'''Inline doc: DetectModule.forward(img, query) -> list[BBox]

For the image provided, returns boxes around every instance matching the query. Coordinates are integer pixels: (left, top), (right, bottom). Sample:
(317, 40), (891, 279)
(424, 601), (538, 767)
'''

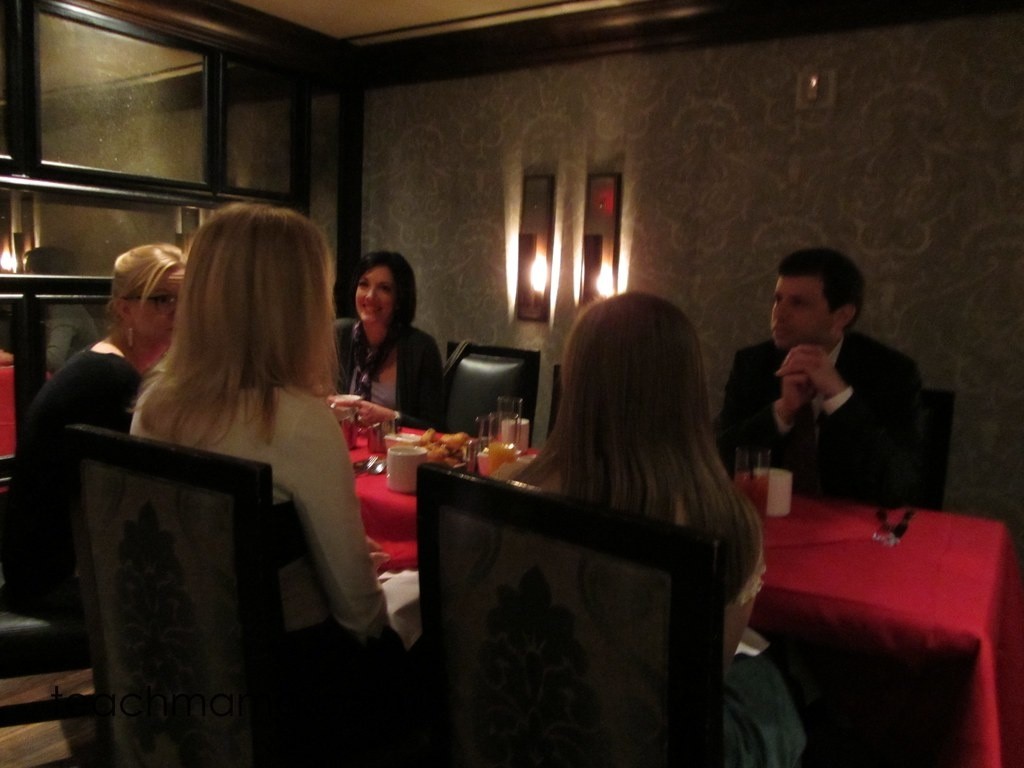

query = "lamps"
(0, 192), (36, 277)
(512, 169), (556, 323)
(576, 173), (622, 313)
(177, 207), (201, 255)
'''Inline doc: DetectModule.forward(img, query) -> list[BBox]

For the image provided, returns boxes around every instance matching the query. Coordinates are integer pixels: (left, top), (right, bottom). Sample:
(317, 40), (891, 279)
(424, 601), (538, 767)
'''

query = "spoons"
(369, 461), (387, 474)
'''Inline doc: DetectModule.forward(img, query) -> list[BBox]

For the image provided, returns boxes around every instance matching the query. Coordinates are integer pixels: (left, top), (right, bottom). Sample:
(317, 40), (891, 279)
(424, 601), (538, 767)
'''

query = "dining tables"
(346, 424), (1024, 768)
(440, 342), (541, 450)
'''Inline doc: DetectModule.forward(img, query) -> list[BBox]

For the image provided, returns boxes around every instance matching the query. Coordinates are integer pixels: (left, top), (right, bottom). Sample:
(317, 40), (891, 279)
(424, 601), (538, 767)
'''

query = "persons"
(710, 246), (925, 507)
(2, 242), (187, 621)
(125, 203), (409, 768)
(332, 249), (448, 433)
(511, 290), (821, 768)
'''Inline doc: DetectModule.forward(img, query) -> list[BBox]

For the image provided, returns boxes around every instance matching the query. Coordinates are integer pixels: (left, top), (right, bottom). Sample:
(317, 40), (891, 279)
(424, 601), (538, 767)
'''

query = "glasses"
(120, 294), (178, 311)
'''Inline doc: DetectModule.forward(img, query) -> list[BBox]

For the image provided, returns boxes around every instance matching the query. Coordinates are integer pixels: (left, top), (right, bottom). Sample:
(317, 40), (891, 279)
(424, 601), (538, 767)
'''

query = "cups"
(479, 397), (529, 453)
(388, 446), (428, 493)
(736, 446), (771, 517)
(327, 394), (363, 450)
(753, 468), (792, 517)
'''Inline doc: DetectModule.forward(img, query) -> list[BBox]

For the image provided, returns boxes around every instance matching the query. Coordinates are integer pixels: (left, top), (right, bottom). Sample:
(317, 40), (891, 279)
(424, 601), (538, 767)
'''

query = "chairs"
(416, 462), (729, 768)
(0, 454), (99, 730)
(64, 422), (285, 768)
(911, 389), (955, 511)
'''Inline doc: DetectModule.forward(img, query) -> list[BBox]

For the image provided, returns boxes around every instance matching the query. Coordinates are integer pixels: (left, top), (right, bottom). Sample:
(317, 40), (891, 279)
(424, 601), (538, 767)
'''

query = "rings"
(330, 403), (335, 410)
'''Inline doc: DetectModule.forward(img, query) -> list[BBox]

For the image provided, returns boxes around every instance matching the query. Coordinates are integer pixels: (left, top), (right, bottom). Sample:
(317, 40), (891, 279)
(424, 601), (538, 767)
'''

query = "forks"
(352, 456), (378, 478)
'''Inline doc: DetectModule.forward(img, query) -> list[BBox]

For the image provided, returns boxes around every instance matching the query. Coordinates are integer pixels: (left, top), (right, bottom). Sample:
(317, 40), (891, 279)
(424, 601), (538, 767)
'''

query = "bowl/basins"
(384, 435), (422, 453)
